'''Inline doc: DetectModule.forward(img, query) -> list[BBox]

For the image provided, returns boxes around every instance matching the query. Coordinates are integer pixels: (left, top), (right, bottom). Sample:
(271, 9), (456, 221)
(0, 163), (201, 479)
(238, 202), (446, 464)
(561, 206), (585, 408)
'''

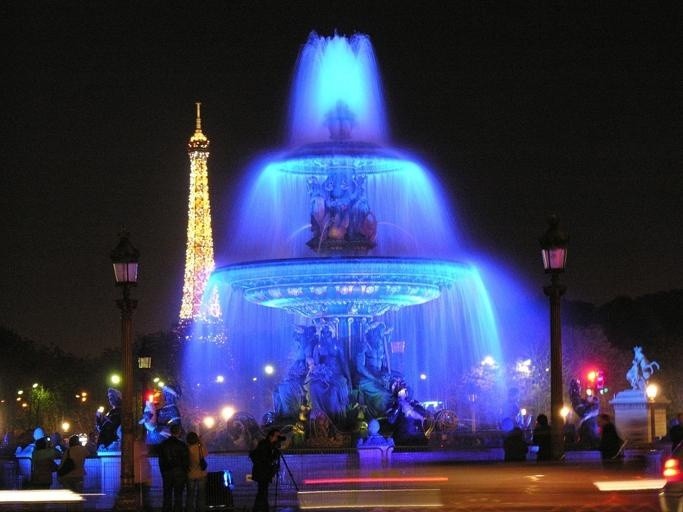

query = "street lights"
(534, 210), (572, 465)
(465, 384), (479, 433)
(134, 340), (152, 442)
(106, 231), (153, 512)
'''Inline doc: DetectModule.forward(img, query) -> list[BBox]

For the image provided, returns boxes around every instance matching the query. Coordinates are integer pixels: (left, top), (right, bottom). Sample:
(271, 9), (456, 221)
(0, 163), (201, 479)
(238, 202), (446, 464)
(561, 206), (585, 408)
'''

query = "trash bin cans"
(207, 473), (233, 508)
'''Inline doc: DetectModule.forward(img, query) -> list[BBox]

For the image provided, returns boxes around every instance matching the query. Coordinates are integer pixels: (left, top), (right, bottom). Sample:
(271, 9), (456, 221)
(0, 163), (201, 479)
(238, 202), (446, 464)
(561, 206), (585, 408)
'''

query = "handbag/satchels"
(199, 457), (208, 471)
(50, 461), (58, 472)
(55, 463), (85, 484)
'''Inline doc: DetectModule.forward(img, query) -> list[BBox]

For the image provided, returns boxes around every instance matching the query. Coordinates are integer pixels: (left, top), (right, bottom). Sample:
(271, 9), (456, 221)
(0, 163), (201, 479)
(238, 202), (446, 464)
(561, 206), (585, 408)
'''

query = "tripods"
(271, 445), (299, 512)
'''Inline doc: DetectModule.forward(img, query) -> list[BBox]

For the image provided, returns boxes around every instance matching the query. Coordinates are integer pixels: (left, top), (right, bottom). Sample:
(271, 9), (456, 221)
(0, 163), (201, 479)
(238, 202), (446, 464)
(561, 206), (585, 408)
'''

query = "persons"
(598, 415), (624, 472)
(571, 378), (597, 437)
(502, 388), (520, 428)
(503, 418), (514, 432)
(158, 424), (189, 512)
(249, 429), (280, 512)
(262, 322), (433, 447)
(503, 427), (529, 462)
(143, 384), (181, 455)
(0, 428), (98, 512)
(96, 388), (123, 453)
(186, 432), (209, 512)
(669, 414), (683, 455)
(533, 415), (551, 462)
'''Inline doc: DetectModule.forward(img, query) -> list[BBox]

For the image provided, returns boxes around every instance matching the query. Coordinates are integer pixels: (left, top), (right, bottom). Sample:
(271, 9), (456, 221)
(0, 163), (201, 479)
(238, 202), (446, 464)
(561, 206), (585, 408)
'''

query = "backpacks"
(172, 440), (191, 469)
(544, 426), (565, 459)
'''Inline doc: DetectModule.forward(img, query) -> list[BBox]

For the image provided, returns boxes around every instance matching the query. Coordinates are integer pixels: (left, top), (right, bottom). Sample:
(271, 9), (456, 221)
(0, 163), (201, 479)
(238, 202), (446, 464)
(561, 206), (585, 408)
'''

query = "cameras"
(275, 435), (288, 444)
